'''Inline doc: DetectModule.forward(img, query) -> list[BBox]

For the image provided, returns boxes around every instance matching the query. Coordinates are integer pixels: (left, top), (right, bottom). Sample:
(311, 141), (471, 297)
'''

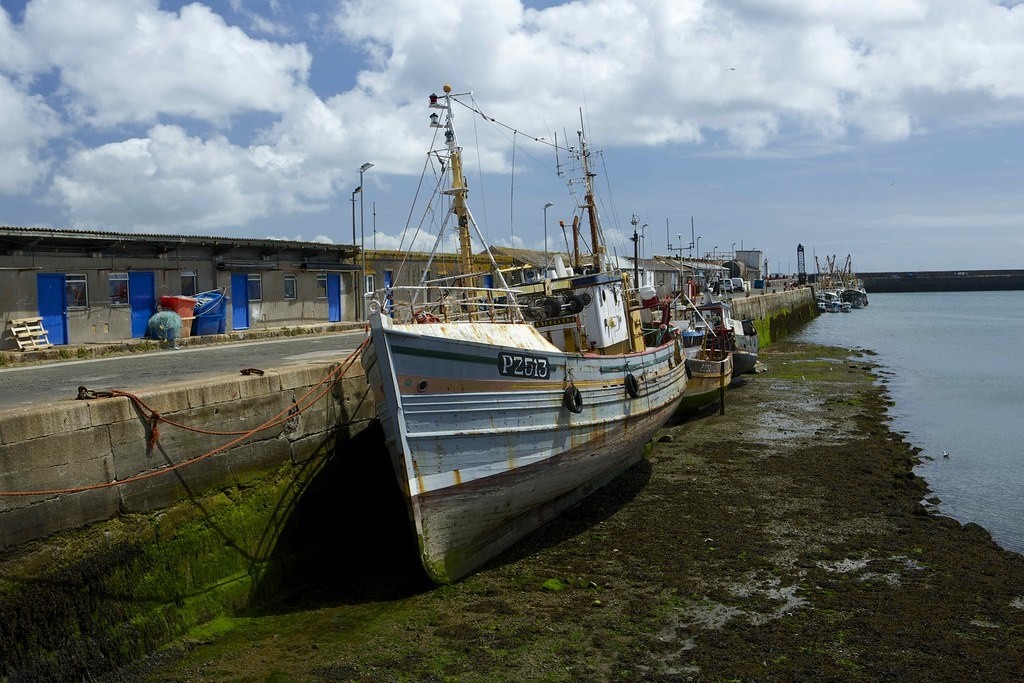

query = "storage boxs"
(672, 320), (690, 330)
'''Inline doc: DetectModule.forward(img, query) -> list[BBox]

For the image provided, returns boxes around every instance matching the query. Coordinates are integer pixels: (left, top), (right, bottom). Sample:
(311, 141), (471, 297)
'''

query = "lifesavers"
(564, 386), (584, 414)
(624, 373), (640, 399)
(685, 359), (692, 380)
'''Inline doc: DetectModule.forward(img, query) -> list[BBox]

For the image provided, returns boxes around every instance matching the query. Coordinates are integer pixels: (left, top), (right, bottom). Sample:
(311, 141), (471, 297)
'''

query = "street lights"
(544, 202), (554, 278)
(360, 163), (375, 321)
(732, 243), (736, 259)
(641, 223), (648, 259)
(353, 186), (364, 321)
(714, 246), (718, 260)
(697, 236), (702, 271)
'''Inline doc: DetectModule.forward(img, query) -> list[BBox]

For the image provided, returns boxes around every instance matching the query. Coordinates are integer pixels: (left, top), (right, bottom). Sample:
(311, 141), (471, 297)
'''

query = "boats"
(815, 255), (868, 313)
(358, 83), (692, 590)
(668, 285), (760, 382)
(666, 316), (735, 424)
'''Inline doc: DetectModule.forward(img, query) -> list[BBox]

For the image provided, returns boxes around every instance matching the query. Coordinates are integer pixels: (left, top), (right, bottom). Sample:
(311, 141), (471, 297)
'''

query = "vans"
(719, 278), (734, 294)
(732, 277), (747, 292)
